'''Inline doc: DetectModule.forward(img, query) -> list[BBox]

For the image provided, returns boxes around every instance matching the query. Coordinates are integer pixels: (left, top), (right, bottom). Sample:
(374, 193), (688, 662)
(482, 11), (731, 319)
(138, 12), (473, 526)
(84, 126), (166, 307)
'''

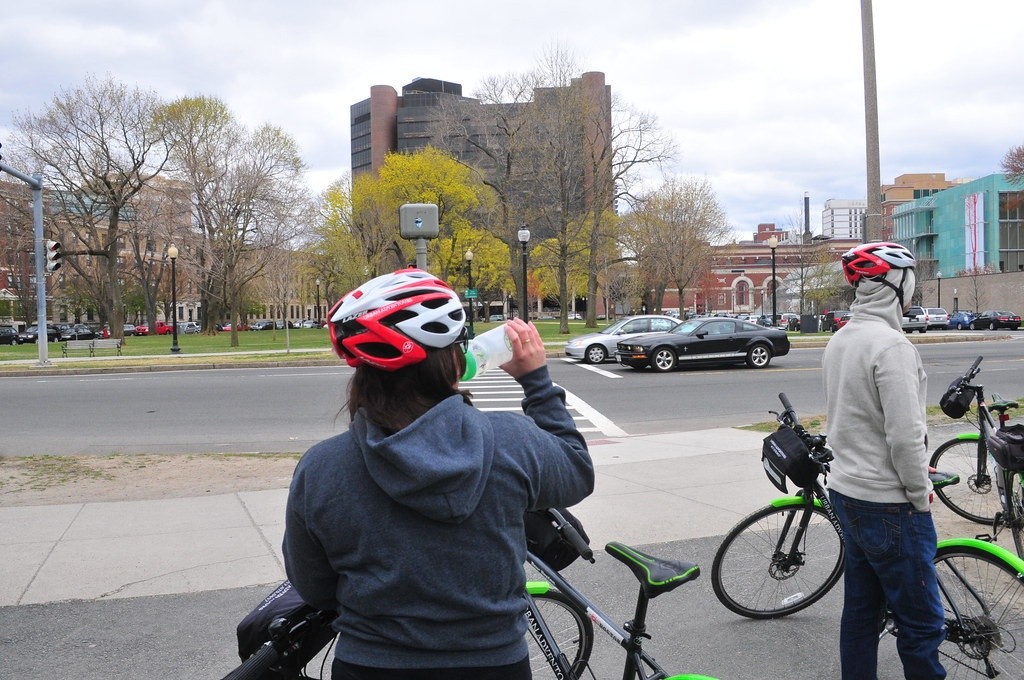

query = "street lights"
(936, 270), (942, 308)
(167, 243), (181, 352)
(123, 303), (127, 323)
(518, 219), (530, 323)
(766, 233), (779, 327)
(315, 278), (320, 330)
(464, 248), (475, 339)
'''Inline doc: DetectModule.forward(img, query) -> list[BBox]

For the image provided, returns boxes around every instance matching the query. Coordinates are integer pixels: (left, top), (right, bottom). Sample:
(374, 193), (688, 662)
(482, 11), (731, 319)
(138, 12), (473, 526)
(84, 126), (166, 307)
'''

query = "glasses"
(452, 326), (468, 353)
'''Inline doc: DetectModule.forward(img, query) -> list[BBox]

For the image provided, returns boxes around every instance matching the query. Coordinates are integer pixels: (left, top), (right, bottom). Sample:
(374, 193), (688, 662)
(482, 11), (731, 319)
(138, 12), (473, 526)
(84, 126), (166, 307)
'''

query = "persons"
(626, 320), (644, 333)
(822, 241), (947, 680)
(281, 266), (594, 680)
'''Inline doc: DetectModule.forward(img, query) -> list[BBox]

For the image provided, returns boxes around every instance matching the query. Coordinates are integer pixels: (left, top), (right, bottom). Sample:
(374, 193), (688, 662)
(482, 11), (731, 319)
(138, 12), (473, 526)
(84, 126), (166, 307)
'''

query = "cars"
(614, 316), (790, 373)
(275, 319), (293, 329)
(672, 310), (852, 334)
(52, 321), (110, 341)
(0, 325), (20, 345)
(970, 310), (1021, 331)
(250, 319), (277, 331)
(482, 314), (503, 322)
(177, 320), (249, 334)
(293, 319), (328, 329)
(537, 312), (605, 320)
(947, 311), (975, 331)
(123, 324), (136, 337)
(135, 321), (174, 336)
(17, 323), (62, 344)
(565, 314), (695, 365)
(923, 307), (949, 331)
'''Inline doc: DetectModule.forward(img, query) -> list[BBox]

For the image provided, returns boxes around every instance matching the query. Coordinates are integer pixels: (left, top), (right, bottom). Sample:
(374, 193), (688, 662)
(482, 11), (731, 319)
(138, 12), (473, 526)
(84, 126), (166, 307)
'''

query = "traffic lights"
(46, 239), (62, 273)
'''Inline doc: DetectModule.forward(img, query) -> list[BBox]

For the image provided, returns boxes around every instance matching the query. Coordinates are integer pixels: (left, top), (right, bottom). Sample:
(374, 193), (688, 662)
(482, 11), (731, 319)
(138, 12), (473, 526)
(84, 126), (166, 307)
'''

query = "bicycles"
(928, 355), (1023, 559)
(524, 506), (719, 680)
(711, 392), (1024, 680)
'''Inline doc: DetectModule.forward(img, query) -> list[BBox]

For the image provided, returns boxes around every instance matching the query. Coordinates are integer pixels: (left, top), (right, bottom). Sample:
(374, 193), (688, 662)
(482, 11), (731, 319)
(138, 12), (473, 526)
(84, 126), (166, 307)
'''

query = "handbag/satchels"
(238, 578), (305, 661)
(988, 424), (1024, 469)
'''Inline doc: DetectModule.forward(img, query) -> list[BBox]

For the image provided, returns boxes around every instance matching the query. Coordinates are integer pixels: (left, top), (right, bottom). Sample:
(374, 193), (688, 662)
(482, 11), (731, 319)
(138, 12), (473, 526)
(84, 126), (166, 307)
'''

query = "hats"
(328, 270), (466, 370)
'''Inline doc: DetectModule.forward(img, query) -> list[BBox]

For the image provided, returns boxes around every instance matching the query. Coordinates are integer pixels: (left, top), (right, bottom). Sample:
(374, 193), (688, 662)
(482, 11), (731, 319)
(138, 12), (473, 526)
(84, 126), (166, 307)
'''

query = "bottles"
(460, 323), (530, 382)
(997, 462), (1005, 490)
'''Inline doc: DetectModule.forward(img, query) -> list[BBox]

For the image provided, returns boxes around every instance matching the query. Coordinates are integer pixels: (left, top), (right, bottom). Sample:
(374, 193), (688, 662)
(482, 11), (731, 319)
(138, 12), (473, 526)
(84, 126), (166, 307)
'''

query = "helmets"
(841, 243), (916, 288)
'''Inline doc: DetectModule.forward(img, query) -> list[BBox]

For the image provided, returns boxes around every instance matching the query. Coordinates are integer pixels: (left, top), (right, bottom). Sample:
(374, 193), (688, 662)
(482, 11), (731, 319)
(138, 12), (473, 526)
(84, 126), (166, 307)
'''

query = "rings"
(522, 338), (530, 343)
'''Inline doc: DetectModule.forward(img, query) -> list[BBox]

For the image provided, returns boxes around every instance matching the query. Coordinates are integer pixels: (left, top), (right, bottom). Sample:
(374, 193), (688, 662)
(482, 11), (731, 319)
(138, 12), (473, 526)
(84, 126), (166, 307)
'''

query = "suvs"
(901, 305), (930, 334)
